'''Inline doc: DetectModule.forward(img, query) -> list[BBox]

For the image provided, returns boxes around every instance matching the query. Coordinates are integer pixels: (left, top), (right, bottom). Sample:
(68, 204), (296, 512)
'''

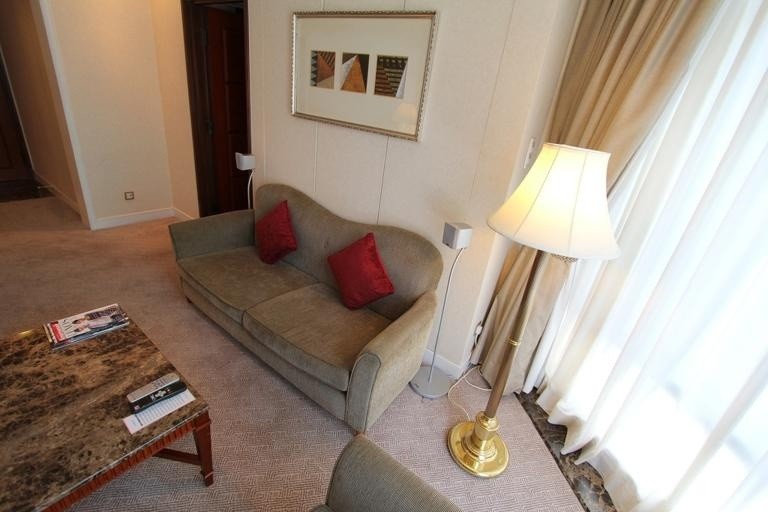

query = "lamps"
(235, 152), (257, 212)
(444, 143), (625, 478)
(406, 221), (474, 398)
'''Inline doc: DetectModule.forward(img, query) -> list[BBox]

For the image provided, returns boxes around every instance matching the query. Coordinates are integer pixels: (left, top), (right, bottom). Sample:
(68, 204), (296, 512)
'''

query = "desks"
(0, 301), (216, 512)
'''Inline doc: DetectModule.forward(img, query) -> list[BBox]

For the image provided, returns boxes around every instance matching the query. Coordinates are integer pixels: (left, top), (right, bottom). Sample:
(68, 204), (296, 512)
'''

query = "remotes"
(129, 382), (186, 412)
(127, 373), (180, 404)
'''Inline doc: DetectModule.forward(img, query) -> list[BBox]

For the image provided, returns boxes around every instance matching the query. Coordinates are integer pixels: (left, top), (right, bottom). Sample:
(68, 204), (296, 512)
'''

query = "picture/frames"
(288, 7), (441, 143)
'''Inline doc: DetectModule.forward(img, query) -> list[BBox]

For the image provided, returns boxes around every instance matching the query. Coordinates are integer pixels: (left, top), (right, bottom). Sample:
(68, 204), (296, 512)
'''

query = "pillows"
(255, 199), (297, 265)
(325, 230), (394, 310)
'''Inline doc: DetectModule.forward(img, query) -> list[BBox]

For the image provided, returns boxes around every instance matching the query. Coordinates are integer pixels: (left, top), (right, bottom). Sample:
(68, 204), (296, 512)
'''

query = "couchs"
(300, 432), (465, 511)
(166, 181), (447, 441)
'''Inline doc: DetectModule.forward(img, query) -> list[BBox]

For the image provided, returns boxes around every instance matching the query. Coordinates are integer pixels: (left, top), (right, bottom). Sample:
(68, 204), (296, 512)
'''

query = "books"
(42, 303), (130, 350)
